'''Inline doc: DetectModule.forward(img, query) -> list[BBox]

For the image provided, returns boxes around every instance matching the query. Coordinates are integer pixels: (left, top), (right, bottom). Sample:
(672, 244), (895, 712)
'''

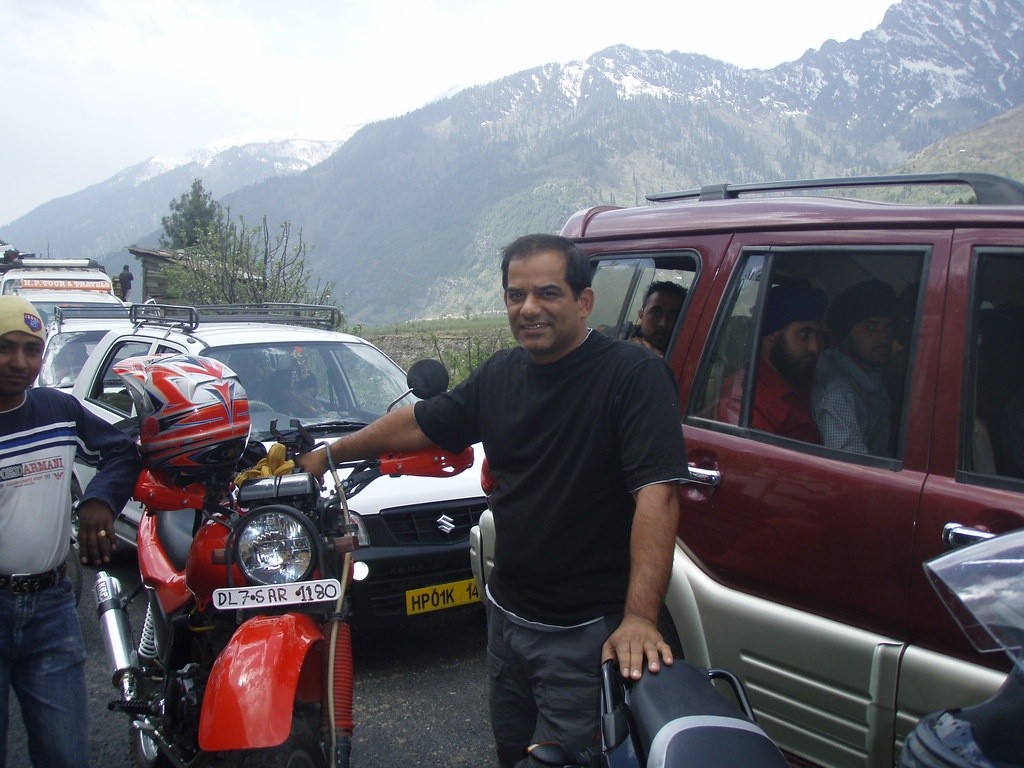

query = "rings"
(98, 530), (105, 536)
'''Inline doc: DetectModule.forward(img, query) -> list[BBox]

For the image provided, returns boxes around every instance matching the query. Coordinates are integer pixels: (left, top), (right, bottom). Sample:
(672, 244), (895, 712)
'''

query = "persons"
(295, 234), (692, 768)
(0, 265), (919, 768)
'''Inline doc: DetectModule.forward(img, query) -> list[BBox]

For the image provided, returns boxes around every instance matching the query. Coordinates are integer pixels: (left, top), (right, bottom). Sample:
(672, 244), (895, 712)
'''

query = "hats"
(761, 286), (827, 335)
(0, 295), (46, 344)
(828, 280), (897, 338)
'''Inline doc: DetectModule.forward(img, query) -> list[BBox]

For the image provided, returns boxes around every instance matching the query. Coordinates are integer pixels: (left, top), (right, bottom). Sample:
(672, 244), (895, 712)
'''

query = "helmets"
(898, 529), (1023, 768)
(112, 353), (252, 486)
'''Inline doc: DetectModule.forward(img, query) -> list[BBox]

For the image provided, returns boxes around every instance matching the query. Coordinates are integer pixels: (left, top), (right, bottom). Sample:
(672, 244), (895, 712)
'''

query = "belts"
(0, 560), (67, 595)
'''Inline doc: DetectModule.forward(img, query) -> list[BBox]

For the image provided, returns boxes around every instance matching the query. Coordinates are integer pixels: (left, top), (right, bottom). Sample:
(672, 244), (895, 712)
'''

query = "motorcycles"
(90, 357), (479, 768)
(594, 522), (1024, 767)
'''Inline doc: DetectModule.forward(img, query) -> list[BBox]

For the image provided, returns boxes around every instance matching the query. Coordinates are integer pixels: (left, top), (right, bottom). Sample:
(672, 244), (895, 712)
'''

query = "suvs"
(0, 259), (161, 396)
(69, 292), (486, 641)
(470, 171), (1024, 768)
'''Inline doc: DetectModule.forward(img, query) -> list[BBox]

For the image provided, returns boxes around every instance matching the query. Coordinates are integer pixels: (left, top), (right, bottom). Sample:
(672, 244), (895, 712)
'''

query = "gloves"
(233, 443), (295, 488)
